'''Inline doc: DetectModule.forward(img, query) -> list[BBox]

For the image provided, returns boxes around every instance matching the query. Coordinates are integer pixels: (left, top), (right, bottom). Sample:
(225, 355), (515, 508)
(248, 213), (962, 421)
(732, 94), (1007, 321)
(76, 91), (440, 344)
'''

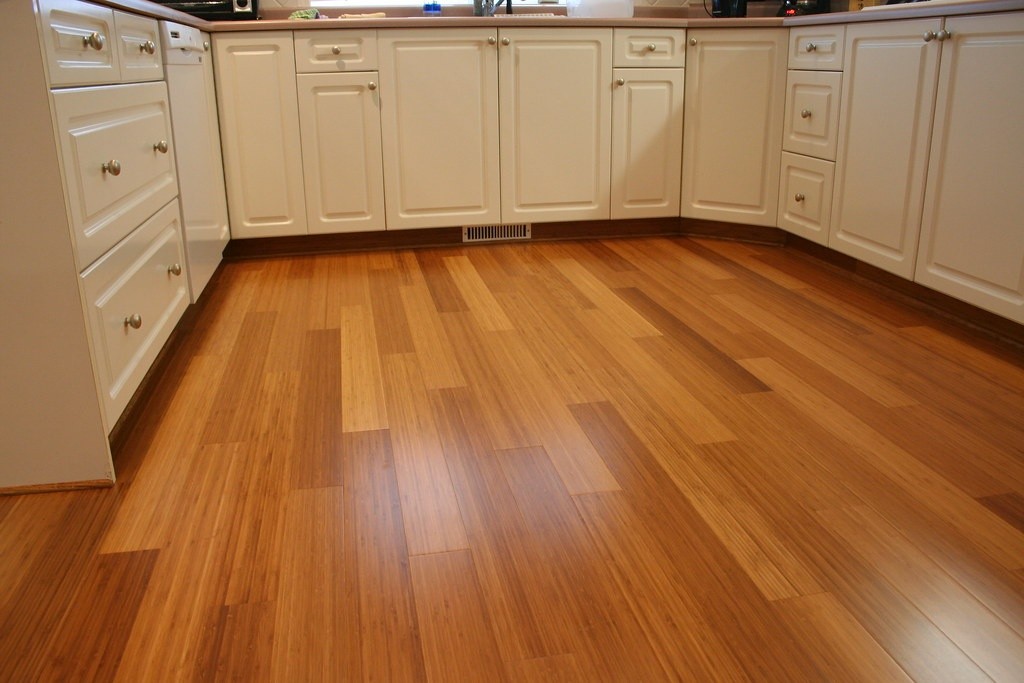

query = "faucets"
(483, 0), (504, 17)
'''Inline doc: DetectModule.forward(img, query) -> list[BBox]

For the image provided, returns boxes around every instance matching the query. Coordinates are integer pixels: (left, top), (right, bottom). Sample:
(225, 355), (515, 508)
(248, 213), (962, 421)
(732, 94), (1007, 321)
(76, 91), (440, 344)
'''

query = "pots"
(703, 0), (749, 18)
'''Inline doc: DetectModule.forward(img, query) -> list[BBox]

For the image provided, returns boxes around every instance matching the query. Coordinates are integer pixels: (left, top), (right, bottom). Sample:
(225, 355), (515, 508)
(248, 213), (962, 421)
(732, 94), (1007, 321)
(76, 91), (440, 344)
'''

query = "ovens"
(152, 0), (259, 23)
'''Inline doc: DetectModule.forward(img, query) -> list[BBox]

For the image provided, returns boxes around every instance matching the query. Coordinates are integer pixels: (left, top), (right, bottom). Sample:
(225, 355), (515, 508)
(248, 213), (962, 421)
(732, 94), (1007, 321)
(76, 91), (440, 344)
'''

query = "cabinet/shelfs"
(377, 25), (612, 250)
(0, 0), (231, 494)
(830, 11), (1024, 346)
(611, 26), (791, 247)
(209, 27), (387, 260)
(777, 25), (846, 261)
